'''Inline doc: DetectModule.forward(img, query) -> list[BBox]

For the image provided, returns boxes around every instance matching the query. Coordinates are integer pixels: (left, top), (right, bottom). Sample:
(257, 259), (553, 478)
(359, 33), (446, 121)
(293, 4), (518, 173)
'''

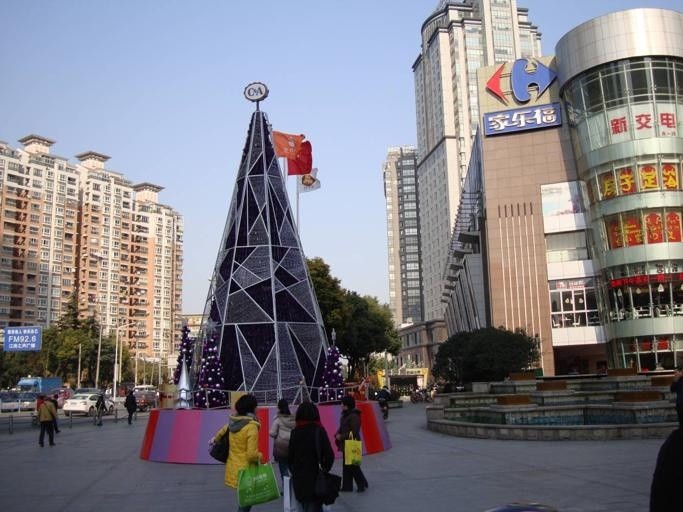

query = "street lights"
(111, 322), (135, 398)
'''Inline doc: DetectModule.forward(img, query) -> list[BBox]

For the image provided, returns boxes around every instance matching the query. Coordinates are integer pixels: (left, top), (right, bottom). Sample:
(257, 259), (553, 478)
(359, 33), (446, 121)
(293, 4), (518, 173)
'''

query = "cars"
(0, 391), (44, 412)
(123, 391), (159, 412)
(62, 393), (114, 417)
(101, 381), (160, 396)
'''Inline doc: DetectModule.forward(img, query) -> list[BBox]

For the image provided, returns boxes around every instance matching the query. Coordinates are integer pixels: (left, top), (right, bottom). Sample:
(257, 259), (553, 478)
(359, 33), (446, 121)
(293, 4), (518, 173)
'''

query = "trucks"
(17, 376), (62, 395)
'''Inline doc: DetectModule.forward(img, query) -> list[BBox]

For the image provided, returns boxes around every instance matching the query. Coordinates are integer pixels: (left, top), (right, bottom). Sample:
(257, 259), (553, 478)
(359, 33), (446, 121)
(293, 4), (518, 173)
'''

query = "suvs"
(44, 387), (73, 408)
(98, 393), (114, 404)
(74, 388), (100, 394)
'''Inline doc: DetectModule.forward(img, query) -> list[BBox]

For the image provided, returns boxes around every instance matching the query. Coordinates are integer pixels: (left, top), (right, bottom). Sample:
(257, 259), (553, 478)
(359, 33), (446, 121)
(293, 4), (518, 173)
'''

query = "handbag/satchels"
(236, 463), (280, 506)
(211, 430), (229, 463)
(344, 440), (363, 465)
(317, 469), (341, 504)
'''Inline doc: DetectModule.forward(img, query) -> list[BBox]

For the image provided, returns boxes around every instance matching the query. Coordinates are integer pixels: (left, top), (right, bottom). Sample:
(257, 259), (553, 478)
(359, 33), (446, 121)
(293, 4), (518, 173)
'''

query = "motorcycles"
(373, 391), (388, 421)
(409, 384), (434, 405)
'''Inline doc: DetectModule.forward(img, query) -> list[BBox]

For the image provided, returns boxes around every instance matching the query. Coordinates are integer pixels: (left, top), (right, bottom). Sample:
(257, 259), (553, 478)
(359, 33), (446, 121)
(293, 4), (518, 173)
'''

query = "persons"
(211, 393), (263, 512)
(270, 398), (298, 497)
(670, 364), (683, 429)
(378, 385), (392, 421)
(35, 392), (60, 447)
(104, 386), (112, 399)
(95, 394), (107, 426)
(334, 396), (369, 496)
(649, 426), (683, 512)
(286, 402), (334, 512)
(124, 390), (137, 425)
(598, 364), (608, 374)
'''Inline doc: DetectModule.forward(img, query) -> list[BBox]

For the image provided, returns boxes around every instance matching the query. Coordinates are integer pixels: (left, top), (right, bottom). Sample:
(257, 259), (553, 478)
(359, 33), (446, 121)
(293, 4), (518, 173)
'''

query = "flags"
(297, 168), (321, 193)
(271, 130), (305, 160)
(286, 140), (312, 175)
